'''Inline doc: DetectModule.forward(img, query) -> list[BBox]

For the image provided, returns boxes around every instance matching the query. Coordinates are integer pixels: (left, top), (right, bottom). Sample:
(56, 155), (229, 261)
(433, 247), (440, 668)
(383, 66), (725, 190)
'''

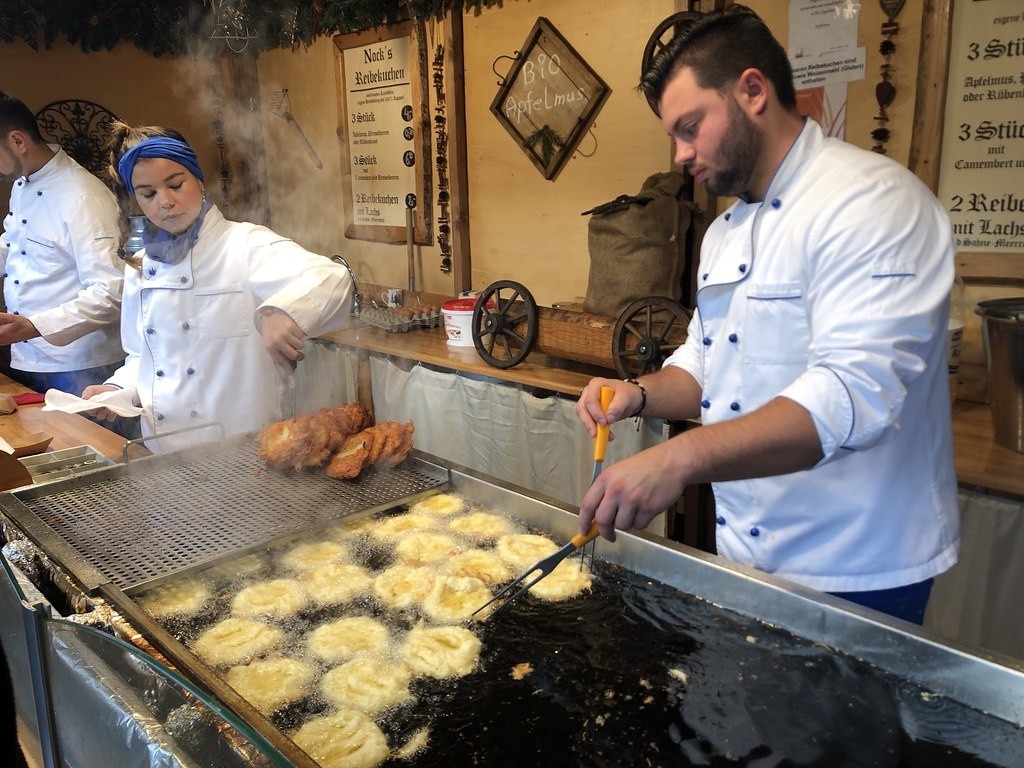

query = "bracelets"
(624, 378), (648, 418)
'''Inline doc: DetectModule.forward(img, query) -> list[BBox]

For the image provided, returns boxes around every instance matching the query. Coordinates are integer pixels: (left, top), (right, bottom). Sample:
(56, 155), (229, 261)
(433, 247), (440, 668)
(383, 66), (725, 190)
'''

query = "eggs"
(393, 303), (441, 320)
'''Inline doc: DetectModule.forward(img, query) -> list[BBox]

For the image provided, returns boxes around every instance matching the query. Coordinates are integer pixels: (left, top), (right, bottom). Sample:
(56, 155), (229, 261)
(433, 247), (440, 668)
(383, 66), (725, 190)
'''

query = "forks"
(473, 521), (602, 624)
(577, 384), (615, 574)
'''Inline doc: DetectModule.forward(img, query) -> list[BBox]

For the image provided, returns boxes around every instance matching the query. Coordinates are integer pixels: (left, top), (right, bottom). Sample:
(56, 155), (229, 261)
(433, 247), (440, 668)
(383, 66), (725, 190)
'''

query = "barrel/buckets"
(441, 299), (494, 346)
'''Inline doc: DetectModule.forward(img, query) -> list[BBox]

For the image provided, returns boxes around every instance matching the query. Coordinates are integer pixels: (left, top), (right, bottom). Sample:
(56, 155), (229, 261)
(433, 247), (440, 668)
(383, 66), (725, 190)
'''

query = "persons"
(577, 5), (961, 627)
(0, 92), (134, 440)
(81, 120), (354, 456)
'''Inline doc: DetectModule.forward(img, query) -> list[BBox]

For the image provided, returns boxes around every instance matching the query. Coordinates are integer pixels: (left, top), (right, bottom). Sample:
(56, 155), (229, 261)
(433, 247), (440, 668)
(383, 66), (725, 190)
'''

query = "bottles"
(947, 270), (966, 408)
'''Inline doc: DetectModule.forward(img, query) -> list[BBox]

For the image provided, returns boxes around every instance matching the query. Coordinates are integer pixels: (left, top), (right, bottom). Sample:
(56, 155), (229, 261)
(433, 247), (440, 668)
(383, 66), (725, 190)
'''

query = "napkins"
(40, 389), (143, 417)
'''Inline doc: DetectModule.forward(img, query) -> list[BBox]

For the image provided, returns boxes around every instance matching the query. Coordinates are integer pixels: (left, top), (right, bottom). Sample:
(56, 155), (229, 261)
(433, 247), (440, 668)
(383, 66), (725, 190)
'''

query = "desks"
(0, 371), (153, 464)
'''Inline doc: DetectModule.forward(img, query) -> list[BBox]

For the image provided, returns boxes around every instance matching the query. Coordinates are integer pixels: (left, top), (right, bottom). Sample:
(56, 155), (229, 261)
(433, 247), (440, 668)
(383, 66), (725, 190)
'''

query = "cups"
(381, 288), (403, 308)
(974, 297), (1024, 453)
